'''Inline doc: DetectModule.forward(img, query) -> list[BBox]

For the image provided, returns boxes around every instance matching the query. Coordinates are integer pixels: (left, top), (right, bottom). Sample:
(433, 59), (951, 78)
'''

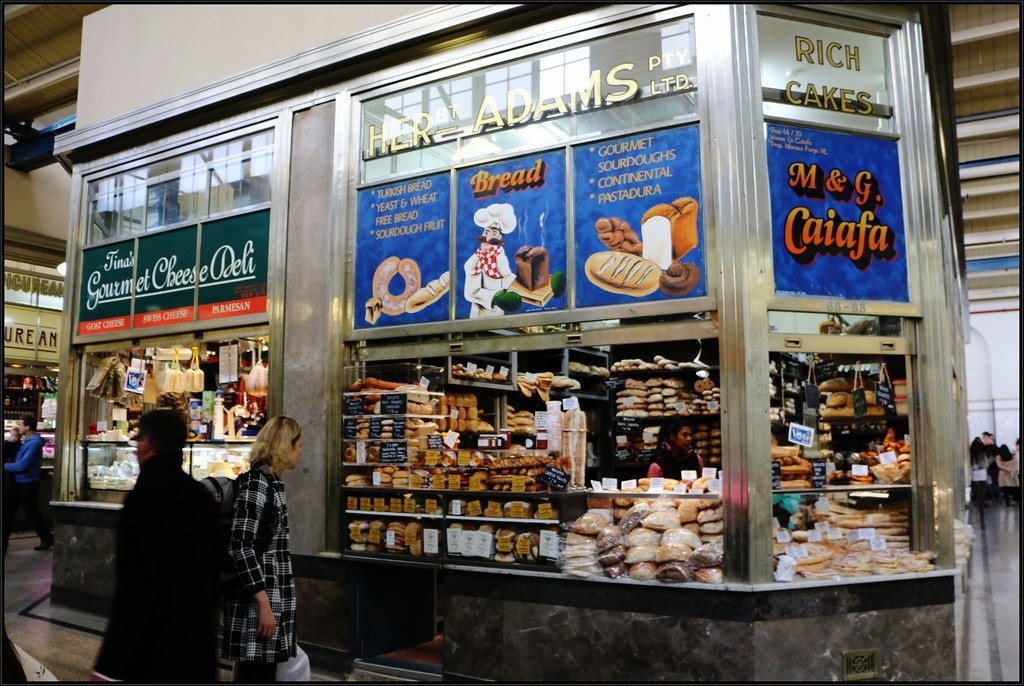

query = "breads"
(342, 354), (726, 584)
(584, 198), (699, 297)
(514, 245), (549, 291)
(365, 256), (450, 325)
(771, 376), (968, 581)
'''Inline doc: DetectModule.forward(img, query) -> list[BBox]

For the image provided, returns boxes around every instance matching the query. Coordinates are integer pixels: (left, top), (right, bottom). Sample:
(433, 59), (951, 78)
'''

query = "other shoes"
(34, 534), (54, 551)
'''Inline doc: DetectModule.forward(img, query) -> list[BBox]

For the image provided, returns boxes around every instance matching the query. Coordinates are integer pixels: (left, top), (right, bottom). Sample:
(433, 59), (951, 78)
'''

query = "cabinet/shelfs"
(336, 320), (912, 567)
(4, 376), (58, 452)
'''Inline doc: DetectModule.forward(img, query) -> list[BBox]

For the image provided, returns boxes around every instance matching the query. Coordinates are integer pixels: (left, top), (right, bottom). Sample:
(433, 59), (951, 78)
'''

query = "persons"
(91, 410), (231, 683)
(2, 415), (55, 554)
(771, 425), (803, 532)
(215, 415), (302, 682)
(647, 418), (703, 479)
(969, 432), (1021, 508)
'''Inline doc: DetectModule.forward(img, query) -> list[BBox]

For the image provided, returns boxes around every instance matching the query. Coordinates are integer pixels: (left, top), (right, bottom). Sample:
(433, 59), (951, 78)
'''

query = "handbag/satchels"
(232, 643), (310, 681)
(198, 470), (274, 574)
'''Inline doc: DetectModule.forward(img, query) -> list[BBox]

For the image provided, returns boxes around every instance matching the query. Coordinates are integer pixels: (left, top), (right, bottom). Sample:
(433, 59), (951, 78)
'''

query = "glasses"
(127, 434), (142, 448)
